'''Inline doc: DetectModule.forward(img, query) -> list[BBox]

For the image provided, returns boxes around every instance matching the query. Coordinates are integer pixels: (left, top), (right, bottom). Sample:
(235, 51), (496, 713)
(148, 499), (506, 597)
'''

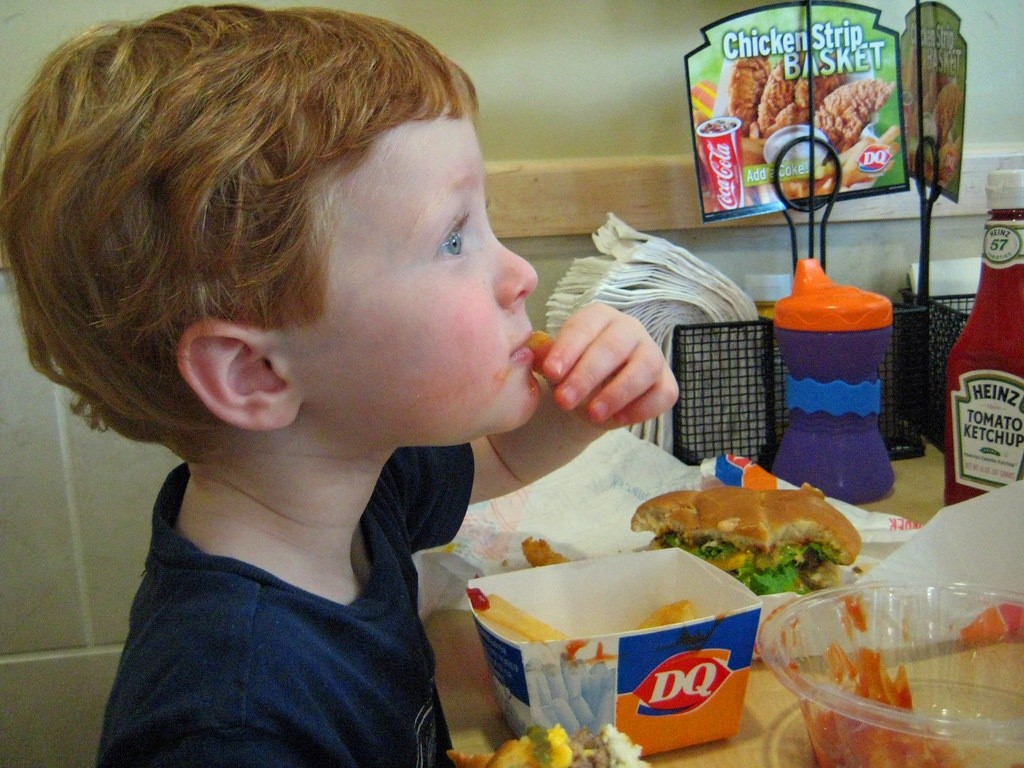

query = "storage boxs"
(465, 547), (763, 756)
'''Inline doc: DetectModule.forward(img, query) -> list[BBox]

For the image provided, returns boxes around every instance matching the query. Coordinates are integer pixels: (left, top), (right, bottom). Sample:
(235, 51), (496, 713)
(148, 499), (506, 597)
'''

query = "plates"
(762, 124), (829, 165)
(918, 112), (938, 145)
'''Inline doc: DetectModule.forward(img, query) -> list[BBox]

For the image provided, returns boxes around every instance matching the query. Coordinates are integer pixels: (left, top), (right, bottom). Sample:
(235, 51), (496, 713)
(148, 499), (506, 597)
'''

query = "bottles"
(945, 155), (1024, 508)
(747, 274), (793, 321)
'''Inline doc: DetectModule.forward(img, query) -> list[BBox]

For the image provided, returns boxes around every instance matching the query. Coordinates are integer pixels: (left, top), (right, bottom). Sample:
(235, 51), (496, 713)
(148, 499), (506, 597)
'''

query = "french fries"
(781, 127), (899, 197)
(476, 595), (566, 644)
(529, 331), (553, 366)
(640, 601), (692, 629)
(523, 537), (569, 567)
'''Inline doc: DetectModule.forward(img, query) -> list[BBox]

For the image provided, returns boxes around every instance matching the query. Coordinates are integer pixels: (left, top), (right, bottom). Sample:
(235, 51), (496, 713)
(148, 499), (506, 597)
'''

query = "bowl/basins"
(757, 581), (1024, 768)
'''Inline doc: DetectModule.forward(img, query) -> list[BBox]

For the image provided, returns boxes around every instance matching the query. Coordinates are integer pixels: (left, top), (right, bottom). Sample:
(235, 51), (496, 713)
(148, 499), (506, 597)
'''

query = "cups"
(770, 259), (894, 505)
(903, 92), (915, 144)
(696, 117), (745, 211)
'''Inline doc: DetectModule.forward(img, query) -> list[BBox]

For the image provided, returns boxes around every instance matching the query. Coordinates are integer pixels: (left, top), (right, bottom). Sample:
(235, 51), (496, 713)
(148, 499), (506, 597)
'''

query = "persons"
(1, 5), (680, 768)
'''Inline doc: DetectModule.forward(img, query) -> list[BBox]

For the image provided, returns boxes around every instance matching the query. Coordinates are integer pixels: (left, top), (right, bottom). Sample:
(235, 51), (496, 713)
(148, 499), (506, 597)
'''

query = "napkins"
(544, 212), (766, 462)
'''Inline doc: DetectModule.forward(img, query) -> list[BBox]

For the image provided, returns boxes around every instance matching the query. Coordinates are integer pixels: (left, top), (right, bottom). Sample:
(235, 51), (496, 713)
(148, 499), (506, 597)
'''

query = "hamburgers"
(631, 487), (860, 596)
(448, 722), (650, 768)
